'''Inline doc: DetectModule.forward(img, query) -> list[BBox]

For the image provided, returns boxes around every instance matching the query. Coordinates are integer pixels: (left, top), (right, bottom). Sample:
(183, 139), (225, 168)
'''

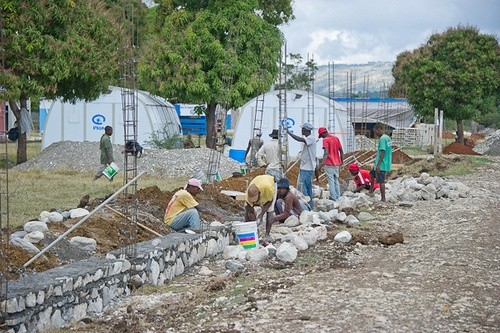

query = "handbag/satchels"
(9, 127), (19, 141)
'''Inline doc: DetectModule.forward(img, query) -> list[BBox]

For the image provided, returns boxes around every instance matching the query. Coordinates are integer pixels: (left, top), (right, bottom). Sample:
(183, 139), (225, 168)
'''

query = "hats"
(269, 129), (279, 138)
(256, 131), (262, 135)
(188, 179), (204, 191)
(348, 164), (359, 170)
(278, 178), (289, 188)
(300, 123), (313, 130)
(248, 184), (259, 202)
(318, 127), (327, 138)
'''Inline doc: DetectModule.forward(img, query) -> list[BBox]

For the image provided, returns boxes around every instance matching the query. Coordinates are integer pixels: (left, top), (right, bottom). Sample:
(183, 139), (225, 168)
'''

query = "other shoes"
(365, 191), (374, 196)
(327, 196), (334, 200)
(190, 227), (200, 233)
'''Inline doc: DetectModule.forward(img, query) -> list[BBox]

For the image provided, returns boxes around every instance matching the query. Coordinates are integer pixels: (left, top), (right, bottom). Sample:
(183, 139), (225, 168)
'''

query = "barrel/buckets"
(240, 165), (248, 176)
(190, 170), (207, 183)
(102, 162), (121, 181)
(207, 174), (215, 183)
(234, 221), (259, 250)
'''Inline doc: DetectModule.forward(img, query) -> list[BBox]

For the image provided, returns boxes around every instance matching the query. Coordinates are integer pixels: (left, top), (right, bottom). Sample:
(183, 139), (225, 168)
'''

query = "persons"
(244, 121), (393, 241)
(163, 179), (204, 230)
(92, 126), (115, 181)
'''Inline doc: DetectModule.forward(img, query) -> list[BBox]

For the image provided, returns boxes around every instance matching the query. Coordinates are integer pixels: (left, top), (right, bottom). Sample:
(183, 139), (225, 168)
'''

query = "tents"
(43, 84), (185, 149)
(328, 98), (420, 131)
(229, 89), (356, 165)
